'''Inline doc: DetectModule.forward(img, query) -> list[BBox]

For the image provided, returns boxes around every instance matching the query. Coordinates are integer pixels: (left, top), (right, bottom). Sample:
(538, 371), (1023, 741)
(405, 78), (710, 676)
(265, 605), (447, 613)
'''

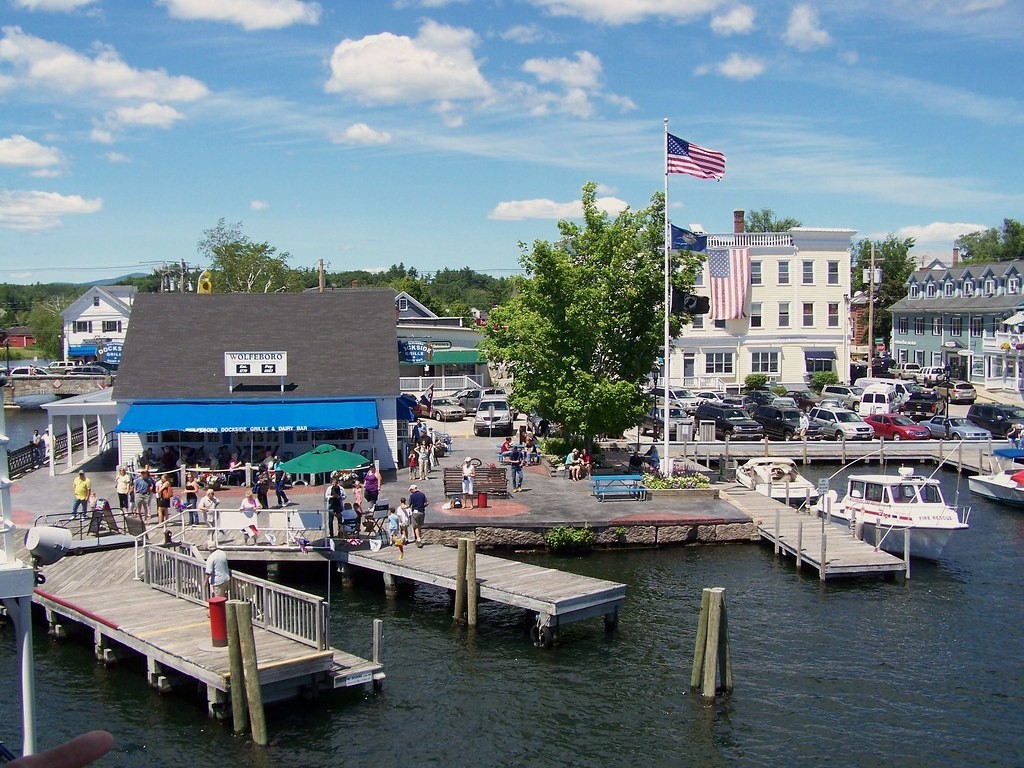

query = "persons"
(114, 444), (291, 618)
(3, 729), (115, 768)
(628, 451), (644, 475)
(749, 409), (754, 419)
(408, 419), (433, 480)
(945, 364), (951, 377)
(1007, 423), (1024, 450)
(648, 449), (660, 471)
(500, 416), (551, 492)
(794, 393), (800, 406)
(388, 485), (429, 548)
(29, 364), (97, 522)
(799, 412), (809, 440)
(461, 456), (476, 509)
(894, 394), (902, 414)
(325, 462), (382, 539)
(934, 398), (946, 415)
(565, 447), (591, 482)
(1009, 329), (1019, 343)
(889, 399), (895, 413)
(644, 445), (658, 455)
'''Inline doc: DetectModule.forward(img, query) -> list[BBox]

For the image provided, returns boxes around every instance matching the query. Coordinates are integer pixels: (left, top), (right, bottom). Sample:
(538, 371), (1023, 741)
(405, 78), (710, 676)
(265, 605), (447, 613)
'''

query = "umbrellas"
(279, 444), (371, 547)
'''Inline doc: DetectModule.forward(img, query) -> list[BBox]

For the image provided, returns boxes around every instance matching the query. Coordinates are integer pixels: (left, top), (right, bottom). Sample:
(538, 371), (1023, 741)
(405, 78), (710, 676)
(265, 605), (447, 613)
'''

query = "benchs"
(557, 467), (570, 479)
(444, 468), (509, 499)
(497, 450), (541, 463)
(590, 482), (648, 502)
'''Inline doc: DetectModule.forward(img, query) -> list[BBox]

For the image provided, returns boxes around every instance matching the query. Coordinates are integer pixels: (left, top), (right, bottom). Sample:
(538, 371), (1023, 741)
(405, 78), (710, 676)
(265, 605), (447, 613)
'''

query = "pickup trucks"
(459, 387), (519, 420)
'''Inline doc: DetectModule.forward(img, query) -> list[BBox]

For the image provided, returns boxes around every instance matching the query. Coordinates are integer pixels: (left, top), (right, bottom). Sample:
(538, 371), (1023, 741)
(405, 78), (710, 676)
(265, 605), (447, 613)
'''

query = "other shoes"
(419, 477), (422, 481)
(425, 477), (429, 480)
(518, 487), (522, 492)
(277, 504), (282, 507)
(283, 501), (290, 506)
(70, 515), (75, 519)
(513, 489), (517, 492)
(417, 541), (421, 547)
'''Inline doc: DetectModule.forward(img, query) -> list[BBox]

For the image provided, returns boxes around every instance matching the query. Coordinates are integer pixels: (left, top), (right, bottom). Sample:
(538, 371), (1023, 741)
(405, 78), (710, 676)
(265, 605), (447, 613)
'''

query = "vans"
(859, 385), (903, 417)
(854, 378), (925, 410)
(70, 366), (117, 375)
(45, 362), (75, 371)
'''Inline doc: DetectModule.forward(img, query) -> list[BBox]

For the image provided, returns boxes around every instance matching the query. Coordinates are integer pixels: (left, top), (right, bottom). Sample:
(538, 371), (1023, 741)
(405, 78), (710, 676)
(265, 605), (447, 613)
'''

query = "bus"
(913, 365), (951, 388)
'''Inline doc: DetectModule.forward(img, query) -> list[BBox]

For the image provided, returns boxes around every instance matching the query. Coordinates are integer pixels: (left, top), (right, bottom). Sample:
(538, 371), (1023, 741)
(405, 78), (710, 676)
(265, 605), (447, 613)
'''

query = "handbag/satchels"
(163, 483), (173, 499)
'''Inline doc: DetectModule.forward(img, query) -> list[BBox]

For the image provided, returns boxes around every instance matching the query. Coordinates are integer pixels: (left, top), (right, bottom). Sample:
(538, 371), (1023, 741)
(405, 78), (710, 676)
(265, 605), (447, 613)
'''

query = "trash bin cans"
(519, 426), (526, 444)
(699, 420), (715, 441)
(676, 420), (693, 441)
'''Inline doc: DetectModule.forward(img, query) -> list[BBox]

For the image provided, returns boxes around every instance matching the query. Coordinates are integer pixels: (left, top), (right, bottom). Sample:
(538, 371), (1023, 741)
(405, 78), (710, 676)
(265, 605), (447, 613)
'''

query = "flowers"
(330, 470), (359, 480)
(198, 472), (226, 486)
(259, 472), (291, 484)
(433, 441), (448, 453)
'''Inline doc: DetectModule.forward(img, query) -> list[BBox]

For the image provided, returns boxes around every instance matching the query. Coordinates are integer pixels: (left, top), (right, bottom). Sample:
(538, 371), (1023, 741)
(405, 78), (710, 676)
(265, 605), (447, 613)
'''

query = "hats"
(408, 484), (417, 490)
(78, 470), (84, 475)
(465, 457), (471, 461)
(208, 541), (216, 550)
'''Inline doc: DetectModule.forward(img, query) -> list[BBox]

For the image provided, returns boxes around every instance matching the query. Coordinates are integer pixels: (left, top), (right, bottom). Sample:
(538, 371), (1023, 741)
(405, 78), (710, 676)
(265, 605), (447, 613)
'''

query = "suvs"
(753, 404), (826, 441)
(822, 384), (866, 411)
(932, 380), (978, 403)
(693, 401), (767, 441)
(888, 362), (922, 381)
(645, 386), (703, 416)
(807, 406), (877, 441)
(967, 402), (1024, 439)
(638, 407), (698, 441)
(473, 400), (515, 435)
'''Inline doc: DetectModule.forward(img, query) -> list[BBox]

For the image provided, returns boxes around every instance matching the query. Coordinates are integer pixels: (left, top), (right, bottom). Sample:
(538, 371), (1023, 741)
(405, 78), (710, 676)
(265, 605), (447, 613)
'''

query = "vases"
(436, 450), (445, 458)
(338, 478), (353, 489)
(269, 479), (276, 489)
(205, 482), (220, 491)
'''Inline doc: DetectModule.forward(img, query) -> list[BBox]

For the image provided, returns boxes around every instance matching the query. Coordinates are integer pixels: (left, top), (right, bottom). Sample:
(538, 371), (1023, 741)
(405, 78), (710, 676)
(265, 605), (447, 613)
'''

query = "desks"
(591, 475), (644, 501)
(498, 445), (540, 458)
(627, 451), (651, 472)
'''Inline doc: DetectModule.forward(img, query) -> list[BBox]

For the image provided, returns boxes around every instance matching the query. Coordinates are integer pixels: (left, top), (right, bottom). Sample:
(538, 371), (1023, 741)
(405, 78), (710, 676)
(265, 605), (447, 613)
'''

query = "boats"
(814, 463), (970, 561)
(735, 456), (841, 512)
(967, 448), (1024, 505)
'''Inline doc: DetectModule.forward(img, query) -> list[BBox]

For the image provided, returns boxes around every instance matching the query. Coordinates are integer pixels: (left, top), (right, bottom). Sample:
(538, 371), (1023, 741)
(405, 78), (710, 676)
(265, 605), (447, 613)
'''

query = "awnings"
(399, 350), (488, 365)
(114, 397), (381, 433)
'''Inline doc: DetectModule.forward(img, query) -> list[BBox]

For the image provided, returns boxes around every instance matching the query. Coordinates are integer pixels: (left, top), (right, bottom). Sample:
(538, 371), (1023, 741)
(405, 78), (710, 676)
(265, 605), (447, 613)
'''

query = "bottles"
(145, 463), (149, 470)
(196, 462), (198, 469)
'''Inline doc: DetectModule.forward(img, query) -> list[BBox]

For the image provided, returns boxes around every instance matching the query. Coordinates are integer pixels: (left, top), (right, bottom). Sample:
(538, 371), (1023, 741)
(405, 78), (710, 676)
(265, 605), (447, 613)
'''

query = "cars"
(11, 368), (51, 376)
(920, 415), (995, 440)
(694, 390), (731, 405)
(447, 388), (476, 401)
(0, 364), (8, 373)
(87, 362), (118, 370)
(421, 396), (467, 419)
(820, 398), (849, 409)
(771, 397), (798, 408)
(725, 395), (758, 413)
(743, 390), (780, 406)
(852, 354), (895, 376)
(778, 390), (825, 412)
(862, 412), (932, 439)
(528, 409), (562, 437)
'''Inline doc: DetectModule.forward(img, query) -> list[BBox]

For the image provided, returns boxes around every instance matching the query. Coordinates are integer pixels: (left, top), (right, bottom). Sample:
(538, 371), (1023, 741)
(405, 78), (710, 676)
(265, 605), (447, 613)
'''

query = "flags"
(421, 385), (433, 419)
(707, 247), (751, 320)
(666, 132), (725, 183)
(240, 525), (365, 554)
(672, 223), (707, 252)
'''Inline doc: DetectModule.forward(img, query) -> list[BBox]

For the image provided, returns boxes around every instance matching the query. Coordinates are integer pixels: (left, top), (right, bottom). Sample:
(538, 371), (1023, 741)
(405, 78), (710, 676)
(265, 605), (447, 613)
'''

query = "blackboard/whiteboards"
(89, 497), (118, 533)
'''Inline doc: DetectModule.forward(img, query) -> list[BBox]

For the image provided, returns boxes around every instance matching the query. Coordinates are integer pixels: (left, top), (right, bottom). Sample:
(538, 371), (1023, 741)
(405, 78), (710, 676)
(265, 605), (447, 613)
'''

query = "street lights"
(943, 364), (953, 441)
(652, 370), (660, 442)
(5, 338), (10, 377)
